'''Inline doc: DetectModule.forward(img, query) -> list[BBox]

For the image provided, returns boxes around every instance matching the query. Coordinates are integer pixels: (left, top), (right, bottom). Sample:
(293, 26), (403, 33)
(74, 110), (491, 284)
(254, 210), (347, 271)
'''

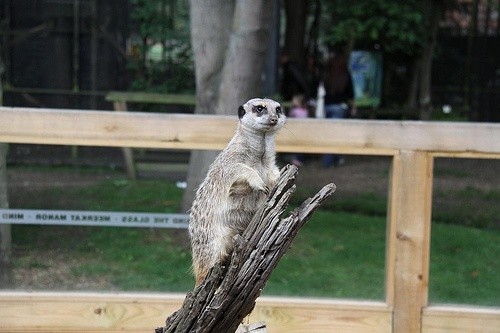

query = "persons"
(316, 49), (357, 167)
(289, 94), (309, 167)
(278, 45), (317, 101)
